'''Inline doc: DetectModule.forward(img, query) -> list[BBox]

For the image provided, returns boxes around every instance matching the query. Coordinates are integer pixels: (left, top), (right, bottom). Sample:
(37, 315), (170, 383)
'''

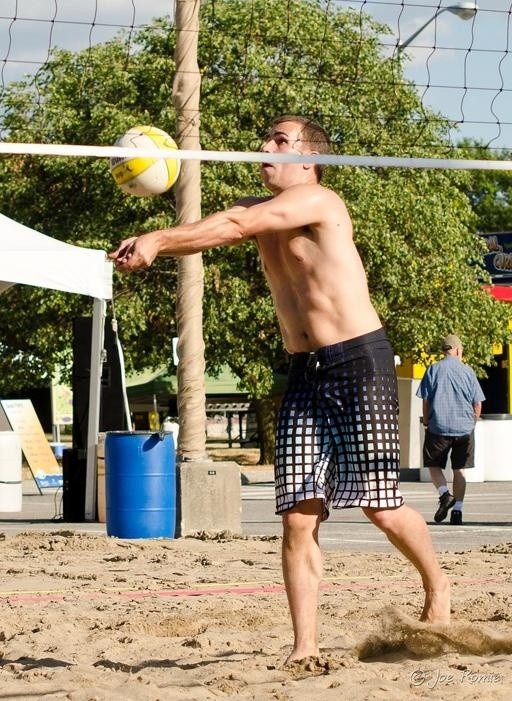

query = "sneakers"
(434, 492), (455, 522)
(450, 510), (462, 524)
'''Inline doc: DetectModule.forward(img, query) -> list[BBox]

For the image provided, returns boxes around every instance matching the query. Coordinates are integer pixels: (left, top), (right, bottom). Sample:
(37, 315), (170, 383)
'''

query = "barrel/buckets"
(97, 432), (107, 522)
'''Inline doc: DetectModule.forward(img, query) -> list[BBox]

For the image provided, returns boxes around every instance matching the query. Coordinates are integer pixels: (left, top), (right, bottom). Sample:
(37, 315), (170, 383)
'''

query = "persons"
(107, 115), (456, 671)
(414, 334), (486, 526)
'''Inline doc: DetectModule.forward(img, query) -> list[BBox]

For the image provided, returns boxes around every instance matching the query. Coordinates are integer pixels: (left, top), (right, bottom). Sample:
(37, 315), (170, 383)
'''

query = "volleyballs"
(110, 124), (181, 196)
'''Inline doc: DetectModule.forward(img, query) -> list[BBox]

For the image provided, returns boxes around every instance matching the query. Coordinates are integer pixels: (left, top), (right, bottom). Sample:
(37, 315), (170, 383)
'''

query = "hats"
(442, 334), (461, 351)
